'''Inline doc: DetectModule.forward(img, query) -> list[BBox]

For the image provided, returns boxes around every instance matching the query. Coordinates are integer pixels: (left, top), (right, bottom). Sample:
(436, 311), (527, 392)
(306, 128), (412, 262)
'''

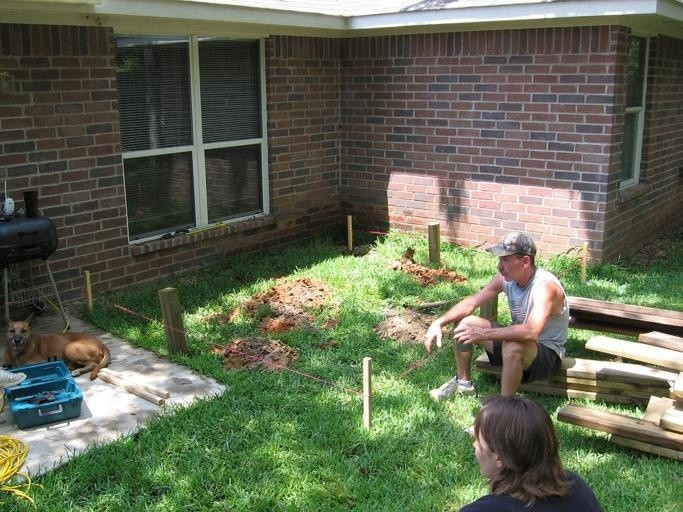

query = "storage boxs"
(1, 360), (83, 430)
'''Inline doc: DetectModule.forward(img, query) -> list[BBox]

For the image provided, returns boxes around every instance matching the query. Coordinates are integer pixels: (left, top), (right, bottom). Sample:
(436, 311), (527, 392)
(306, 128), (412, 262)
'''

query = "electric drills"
(19, 393), (55, 403)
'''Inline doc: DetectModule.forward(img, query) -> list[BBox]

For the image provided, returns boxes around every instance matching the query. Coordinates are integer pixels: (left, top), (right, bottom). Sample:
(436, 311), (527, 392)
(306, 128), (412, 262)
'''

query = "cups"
(457, 342), (472, 351)
(23, 191), (39, 218)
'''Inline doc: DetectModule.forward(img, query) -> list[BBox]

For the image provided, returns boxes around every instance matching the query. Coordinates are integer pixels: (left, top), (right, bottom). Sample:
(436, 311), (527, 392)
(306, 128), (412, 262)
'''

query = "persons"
(419, 231), (570, 434)
(457, 396), (602, 511)
(0, 366), (27, 414)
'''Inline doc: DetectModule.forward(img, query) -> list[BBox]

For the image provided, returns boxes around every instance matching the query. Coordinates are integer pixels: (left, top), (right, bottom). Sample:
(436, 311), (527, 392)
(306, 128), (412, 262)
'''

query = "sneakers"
(430, 374), (476, 399)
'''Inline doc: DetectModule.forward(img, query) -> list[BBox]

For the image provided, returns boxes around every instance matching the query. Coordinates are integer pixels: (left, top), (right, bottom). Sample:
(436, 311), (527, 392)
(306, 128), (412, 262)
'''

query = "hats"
(484, 231), (537, 258)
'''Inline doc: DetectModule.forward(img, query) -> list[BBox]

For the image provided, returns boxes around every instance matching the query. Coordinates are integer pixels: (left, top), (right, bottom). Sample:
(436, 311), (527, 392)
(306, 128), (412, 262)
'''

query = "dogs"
(0, 311), (111, 381)
